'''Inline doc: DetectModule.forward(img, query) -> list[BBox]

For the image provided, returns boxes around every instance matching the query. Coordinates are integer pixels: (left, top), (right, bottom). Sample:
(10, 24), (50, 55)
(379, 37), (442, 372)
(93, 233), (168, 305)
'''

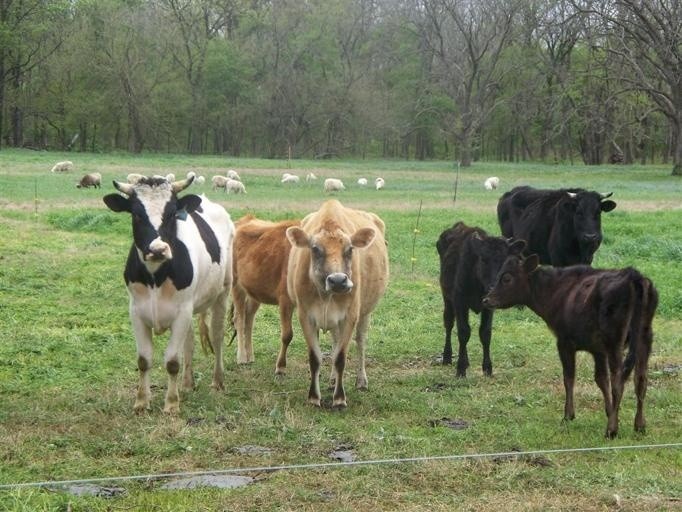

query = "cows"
(435, 221), (529, 380)
(226, 213), (320, 376)
(286, 198), (391, 410)
(481, 253), (660, 441)
(101, 174), (237, 417)
(497, 185), (617, 269)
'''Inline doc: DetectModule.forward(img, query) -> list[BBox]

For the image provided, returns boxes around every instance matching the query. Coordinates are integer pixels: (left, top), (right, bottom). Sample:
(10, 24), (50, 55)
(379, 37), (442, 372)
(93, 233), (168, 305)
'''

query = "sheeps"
(51, 161), (74, 172)
(75, 172), (102, 189)
(324, 178), (346, 193)
(358, 178), (368, 186)
(374, 178), (385, 191)
(484, 176), (499, 191)
(126, 169), (248, 196)
(280, 174), (318, 184)
